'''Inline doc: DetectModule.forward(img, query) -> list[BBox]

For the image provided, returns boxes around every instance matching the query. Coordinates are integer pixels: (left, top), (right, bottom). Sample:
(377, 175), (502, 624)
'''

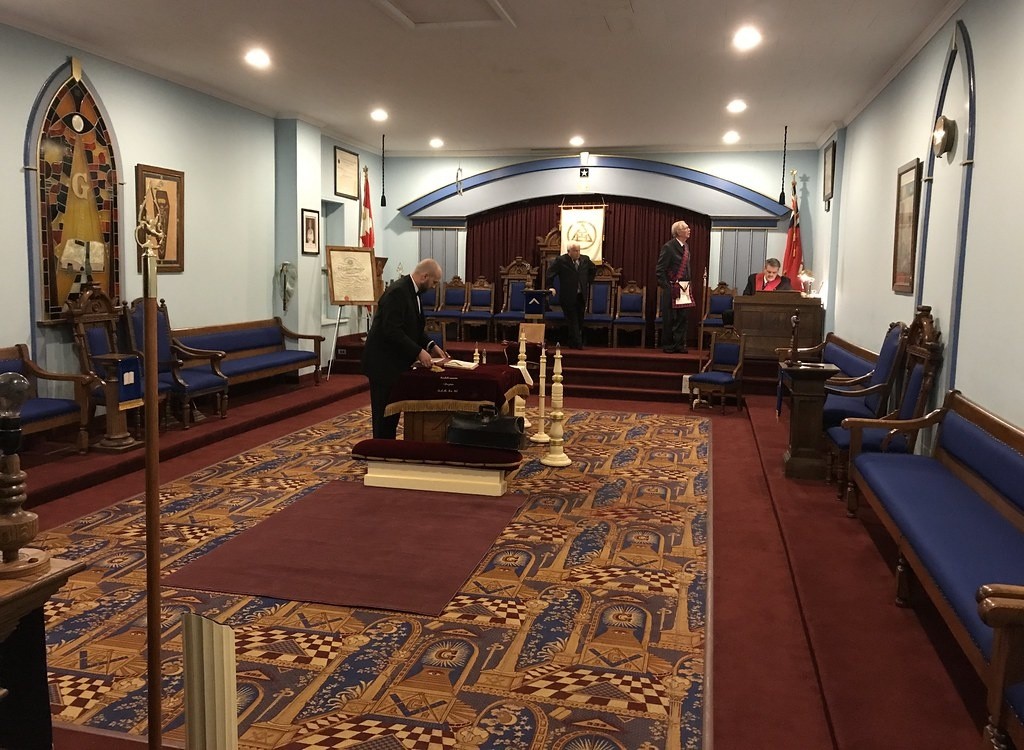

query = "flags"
(361, 172), (376, 249)
(781, 185), (808, 293)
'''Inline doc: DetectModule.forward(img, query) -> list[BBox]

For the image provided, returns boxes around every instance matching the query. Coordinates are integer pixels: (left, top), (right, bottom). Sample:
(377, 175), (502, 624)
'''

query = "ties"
(416, 291), (424, 314)
(573, 259), (581, 292)
(682, 245), (685, 251)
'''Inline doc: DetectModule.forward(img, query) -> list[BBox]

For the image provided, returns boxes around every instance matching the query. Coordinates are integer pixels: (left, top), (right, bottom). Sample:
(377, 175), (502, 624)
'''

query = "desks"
(0, 558), (87, 750)
(90, 353), (145, 455)
(733, 290), (823, 363)
(779, 361), (841, 465)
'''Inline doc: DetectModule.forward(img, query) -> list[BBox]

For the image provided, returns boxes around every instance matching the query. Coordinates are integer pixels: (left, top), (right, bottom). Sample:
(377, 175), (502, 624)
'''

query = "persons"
(359, 259), (446, 440)
(545, 242), (596, 350)
(656, 221), (696, 354)
(743, 258), (792, 297)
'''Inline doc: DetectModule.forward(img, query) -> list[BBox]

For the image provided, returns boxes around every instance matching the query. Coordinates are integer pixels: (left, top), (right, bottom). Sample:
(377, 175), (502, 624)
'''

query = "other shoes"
(576, 343), (583, 350)
(568, 342), (576, 349)
(676, 343), (688, 354)
(663, 342), (675, 353)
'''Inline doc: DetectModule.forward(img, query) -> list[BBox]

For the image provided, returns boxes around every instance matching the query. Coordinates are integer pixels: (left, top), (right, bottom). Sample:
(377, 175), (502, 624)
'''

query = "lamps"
(796, 268), (815, 299)
(381, 135), (386, 206)
(779, 126), (787, 205)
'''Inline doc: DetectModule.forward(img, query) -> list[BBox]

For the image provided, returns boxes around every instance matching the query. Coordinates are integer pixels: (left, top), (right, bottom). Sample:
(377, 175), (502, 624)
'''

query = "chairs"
(417, 220), (748, 416)
(0, 285), (228, 457)
(821, 304), (946, 502)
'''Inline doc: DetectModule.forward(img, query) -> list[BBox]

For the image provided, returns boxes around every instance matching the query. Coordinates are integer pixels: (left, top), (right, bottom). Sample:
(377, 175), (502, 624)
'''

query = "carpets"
(159, 480), (532, 617)
(24, 398), (714, 750)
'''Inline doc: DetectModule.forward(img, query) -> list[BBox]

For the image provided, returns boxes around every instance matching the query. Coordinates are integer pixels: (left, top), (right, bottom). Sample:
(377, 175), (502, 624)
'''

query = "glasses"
(572, 249), (580, 253)
(678, 226), (689, 231)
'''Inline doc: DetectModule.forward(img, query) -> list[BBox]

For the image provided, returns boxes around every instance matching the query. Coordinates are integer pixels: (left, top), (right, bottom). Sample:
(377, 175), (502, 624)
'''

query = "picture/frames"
(325, 244), (379, 306)
(135, 163), (185, 274)
(301, 208), (320, 255)
(822, 140), (836, 202)
(334, 145), (360, 201)
(891, 157), (921, 294)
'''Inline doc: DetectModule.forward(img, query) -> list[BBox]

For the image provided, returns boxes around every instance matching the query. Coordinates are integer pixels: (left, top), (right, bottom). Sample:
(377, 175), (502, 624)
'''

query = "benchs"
(171, 314), (325, 418)
(773, 331), (871, 414)
(840, 387), (1024, 750)
